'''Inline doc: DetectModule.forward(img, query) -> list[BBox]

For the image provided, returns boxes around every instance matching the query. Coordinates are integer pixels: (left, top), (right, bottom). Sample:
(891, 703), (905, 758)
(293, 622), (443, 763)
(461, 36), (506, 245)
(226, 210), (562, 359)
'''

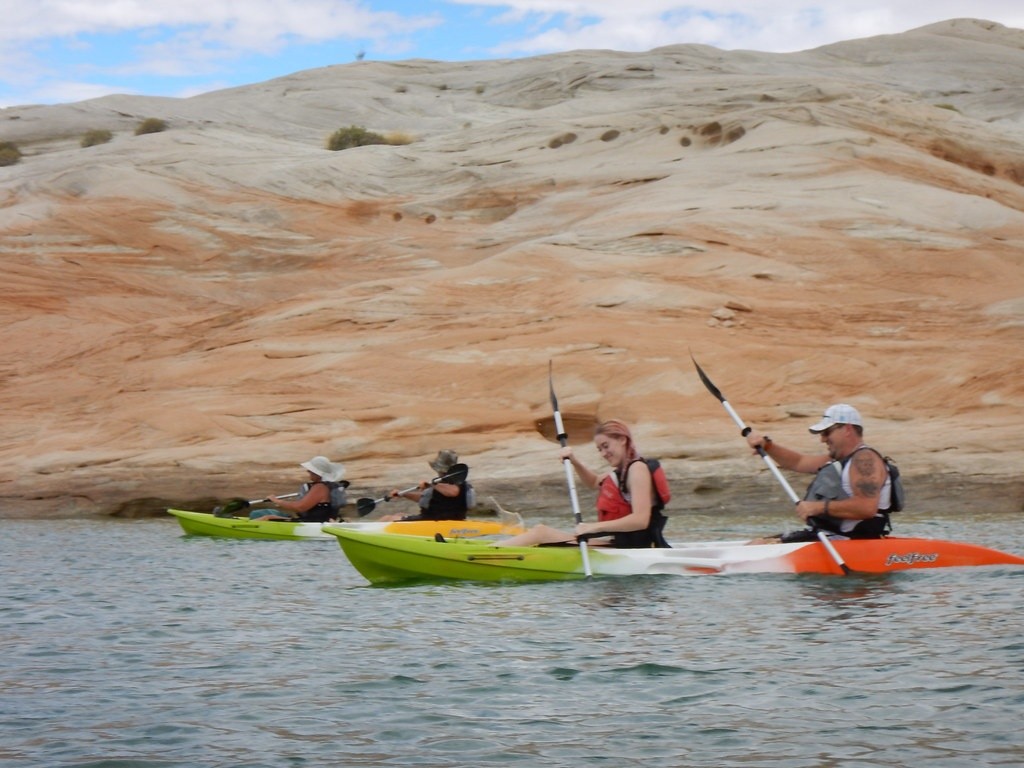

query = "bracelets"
(824, 498), (829, 514)
(763, 435), (772, 452)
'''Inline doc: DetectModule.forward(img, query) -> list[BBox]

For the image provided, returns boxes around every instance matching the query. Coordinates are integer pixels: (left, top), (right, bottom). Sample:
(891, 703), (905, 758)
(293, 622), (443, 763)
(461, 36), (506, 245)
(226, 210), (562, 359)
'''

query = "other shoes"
(435, 533), (448, 542)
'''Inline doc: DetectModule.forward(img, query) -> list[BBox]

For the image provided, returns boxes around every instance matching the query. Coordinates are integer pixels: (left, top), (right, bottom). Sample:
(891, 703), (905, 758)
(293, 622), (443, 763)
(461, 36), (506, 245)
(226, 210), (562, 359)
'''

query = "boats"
(168, 497), (530, 541)
(320, 526), (1024, 585)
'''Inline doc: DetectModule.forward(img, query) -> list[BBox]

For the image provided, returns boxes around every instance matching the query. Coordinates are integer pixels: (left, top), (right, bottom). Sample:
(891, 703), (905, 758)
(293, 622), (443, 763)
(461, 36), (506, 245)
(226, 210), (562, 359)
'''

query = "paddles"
(217, 478), (353, 516)
(546, 354), (597, 583)
(683, 341), (860, 578)
(354, 461), (471, 519)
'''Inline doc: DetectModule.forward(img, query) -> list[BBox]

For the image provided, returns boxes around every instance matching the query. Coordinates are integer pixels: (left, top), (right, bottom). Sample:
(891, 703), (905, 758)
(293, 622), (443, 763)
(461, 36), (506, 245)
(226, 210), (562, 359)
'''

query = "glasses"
(822, 423), (844, 435)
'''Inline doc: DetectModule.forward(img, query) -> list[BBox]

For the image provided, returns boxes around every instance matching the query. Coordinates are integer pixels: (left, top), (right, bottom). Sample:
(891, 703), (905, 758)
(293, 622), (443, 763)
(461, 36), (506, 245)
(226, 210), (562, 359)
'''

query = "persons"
(435, 420), (661, 548)
(266, 457), (333, 524)
(748, 404), (891, 546)
(376, 450), (468, 522)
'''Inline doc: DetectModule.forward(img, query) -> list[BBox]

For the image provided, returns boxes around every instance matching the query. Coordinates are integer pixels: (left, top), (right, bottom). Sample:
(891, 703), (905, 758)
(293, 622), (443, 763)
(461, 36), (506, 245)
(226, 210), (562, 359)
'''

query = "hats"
(427, 449), (458, 472)
(300, 457), (346, 483)
(809, 403), (863, 434)
